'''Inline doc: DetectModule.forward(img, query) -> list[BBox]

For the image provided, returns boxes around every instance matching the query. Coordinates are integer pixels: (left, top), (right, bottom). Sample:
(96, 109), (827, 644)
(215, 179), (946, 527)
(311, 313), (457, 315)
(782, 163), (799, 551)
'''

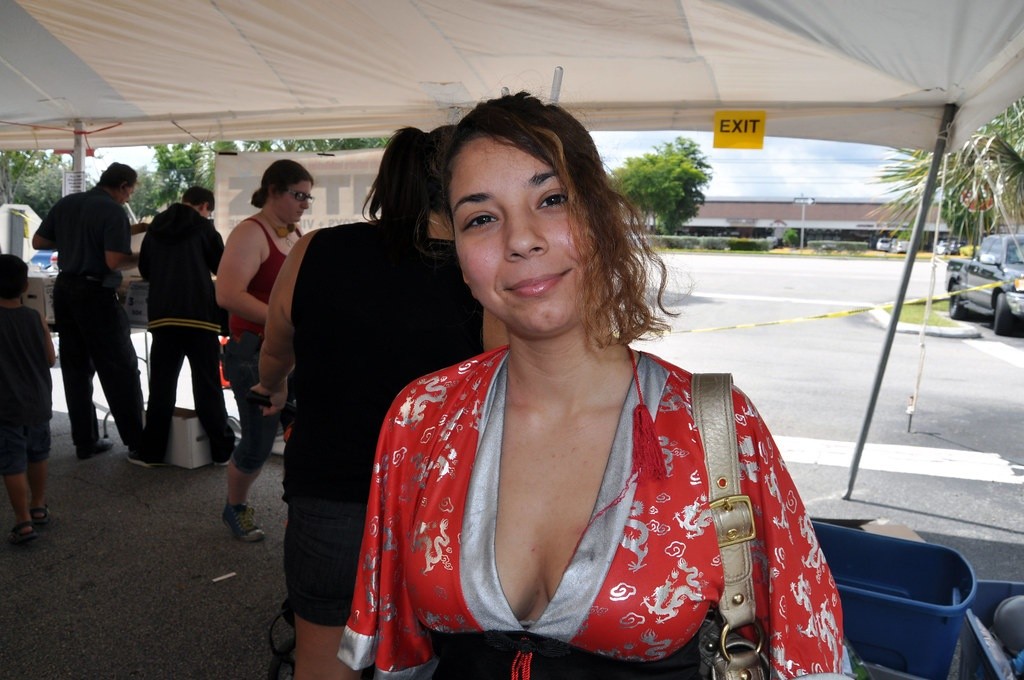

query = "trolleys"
(810, 520), (980, 680)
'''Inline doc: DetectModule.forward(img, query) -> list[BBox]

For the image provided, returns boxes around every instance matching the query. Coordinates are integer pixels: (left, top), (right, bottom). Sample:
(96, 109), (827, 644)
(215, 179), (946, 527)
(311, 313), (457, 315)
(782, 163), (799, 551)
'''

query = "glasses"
(285, 188), (315, 204)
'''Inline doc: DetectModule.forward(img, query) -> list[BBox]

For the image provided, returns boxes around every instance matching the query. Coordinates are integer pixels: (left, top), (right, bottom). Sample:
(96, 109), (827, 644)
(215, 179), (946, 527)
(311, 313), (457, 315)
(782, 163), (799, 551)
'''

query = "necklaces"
(260, 214), (296, 246)
(429, 217), (454, 236)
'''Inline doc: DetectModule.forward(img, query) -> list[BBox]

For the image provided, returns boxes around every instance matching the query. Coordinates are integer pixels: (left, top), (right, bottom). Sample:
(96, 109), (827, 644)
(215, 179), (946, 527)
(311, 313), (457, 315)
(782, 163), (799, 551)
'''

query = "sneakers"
(222, 498), (265, 543)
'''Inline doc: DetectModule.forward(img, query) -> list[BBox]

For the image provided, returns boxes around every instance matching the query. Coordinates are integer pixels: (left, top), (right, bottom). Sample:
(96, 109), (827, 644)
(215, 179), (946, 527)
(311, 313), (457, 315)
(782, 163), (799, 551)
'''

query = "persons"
(337, 92), (854, 679)
(125, 187), (237, 469)
(32, 161), (151, 460)
(250, 124), (508, 680)
(1, 253), (57, 541)
(215, 159), (316, 540)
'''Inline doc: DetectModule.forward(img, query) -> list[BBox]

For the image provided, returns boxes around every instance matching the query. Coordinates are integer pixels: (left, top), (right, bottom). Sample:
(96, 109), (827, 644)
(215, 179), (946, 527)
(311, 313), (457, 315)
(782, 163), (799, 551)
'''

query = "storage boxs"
(957, 580), (1024, 680)
(21, 273), (58, 324)
(124, 279), (149, 324)
(810, 521), (977, 680)
(810, 517), (926, 542)
(163, 407), (212, 470)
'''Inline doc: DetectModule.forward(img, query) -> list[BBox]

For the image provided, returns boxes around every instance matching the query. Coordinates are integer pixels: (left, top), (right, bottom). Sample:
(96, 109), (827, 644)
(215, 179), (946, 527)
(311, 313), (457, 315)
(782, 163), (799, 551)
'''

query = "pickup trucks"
(944, 234), (1024, 336)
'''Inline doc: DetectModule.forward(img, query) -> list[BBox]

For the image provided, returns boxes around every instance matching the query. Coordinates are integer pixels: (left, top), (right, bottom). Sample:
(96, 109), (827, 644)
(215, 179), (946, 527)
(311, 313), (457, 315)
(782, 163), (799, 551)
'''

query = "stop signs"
(961, 179), (995, 213)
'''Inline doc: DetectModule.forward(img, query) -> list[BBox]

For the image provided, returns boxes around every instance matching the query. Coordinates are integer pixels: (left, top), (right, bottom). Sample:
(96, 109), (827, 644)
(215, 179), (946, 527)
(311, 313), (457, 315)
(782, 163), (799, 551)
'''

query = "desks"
(45, 320), (150, 438)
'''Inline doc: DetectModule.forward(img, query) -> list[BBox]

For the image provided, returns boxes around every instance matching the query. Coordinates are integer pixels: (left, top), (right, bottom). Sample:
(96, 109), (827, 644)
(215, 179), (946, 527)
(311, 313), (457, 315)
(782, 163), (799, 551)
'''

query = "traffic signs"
(795, 198), (814, 204)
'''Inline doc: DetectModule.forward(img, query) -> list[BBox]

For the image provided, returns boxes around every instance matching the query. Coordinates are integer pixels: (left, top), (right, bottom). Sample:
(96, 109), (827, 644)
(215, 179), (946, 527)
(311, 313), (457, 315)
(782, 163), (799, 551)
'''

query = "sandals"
(5, 520), (38, 545)
(29, 503), (52, 525)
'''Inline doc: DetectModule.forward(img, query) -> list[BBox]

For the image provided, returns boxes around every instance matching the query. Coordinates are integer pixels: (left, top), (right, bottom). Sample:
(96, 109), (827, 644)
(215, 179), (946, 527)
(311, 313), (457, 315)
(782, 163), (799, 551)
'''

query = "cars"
(892, 239), (910, 253)
(937, 240), (960, 255)
(876, 238), (892, 251)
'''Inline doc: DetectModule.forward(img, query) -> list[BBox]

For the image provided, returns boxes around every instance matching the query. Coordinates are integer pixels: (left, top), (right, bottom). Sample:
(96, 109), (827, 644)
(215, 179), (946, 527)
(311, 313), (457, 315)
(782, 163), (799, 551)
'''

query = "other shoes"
(75, 438), (113, 460)
(126, 452), (168, 469)
(213, 453), (233, 466)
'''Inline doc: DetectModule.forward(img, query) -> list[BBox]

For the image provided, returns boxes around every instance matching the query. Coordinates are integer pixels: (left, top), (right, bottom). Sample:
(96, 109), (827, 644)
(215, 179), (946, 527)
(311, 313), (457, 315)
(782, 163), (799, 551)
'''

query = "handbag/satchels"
(690, 370), (777, 680)
